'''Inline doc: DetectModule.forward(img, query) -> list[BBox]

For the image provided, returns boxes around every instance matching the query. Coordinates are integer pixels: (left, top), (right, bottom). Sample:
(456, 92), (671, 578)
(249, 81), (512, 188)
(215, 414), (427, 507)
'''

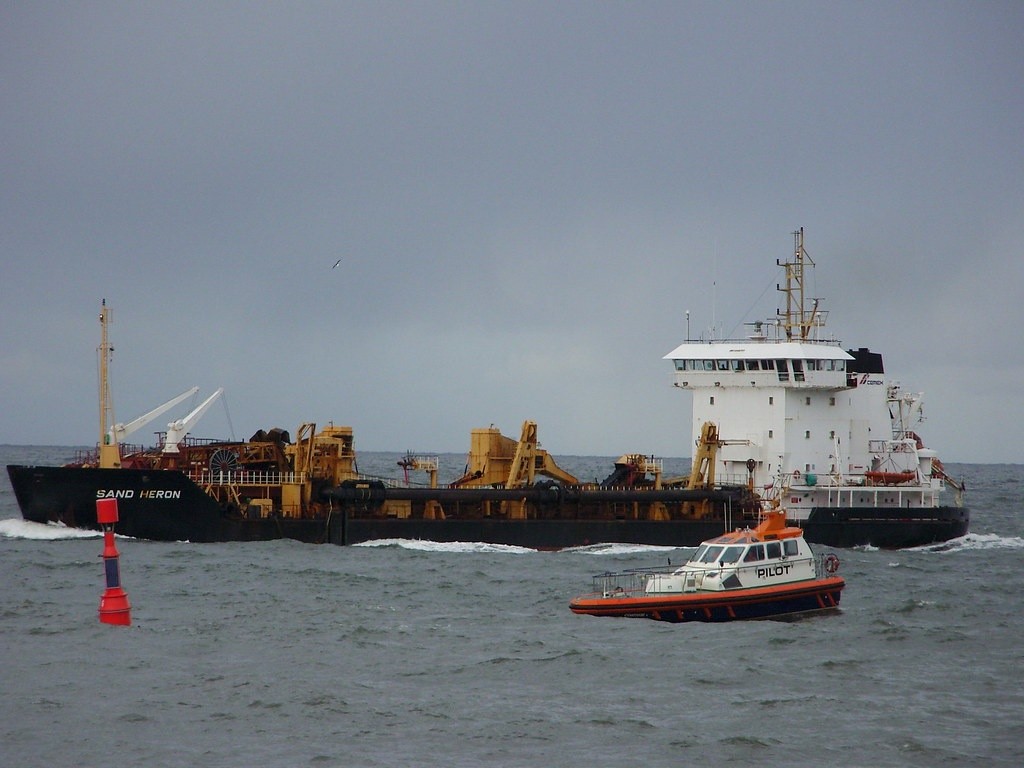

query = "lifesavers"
(794, 470), (800, 479)
(825, 556), (838, 573)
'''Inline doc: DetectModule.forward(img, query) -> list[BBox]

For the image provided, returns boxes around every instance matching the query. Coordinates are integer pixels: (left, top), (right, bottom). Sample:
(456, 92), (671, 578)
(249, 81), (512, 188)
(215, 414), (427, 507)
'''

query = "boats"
(863, 467), (916, 485)
(4, 225), (971, 550)
(568, 507), (847, 623)
